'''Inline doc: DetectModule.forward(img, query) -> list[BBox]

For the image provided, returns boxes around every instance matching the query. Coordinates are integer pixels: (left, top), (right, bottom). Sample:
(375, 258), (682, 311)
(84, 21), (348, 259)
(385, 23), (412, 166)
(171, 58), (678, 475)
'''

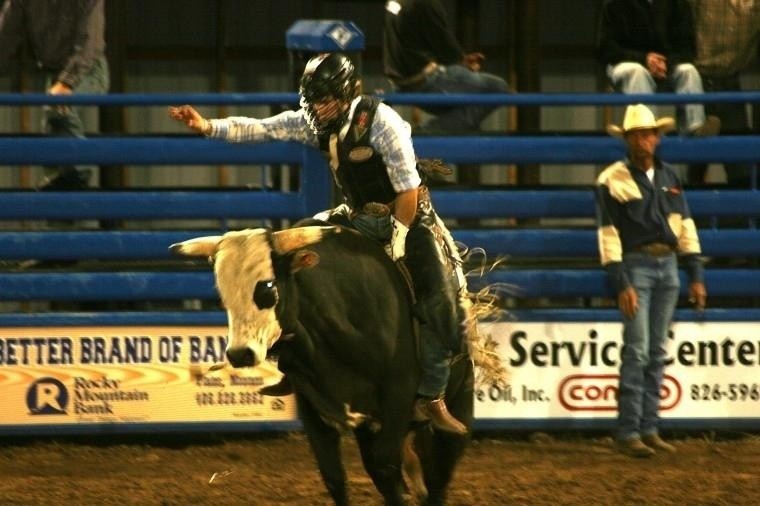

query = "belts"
(633, 242), (678, 257)
(357, 189), (425, 217)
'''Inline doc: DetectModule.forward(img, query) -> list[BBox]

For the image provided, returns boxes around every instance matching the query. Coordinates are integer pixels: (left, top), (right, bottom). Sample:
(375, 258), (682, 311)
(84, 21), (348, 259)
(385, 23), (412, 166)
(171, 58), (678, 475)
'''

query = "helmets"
(298, 52), (361, 136)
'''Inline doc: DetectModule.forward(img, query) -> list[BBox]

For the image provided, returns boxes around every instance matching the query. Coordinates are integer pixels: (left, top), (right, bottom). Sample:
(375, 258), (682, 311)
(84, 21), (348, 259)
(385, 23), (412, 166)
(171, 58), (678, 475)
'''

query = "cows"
(167, 210), (487, 506)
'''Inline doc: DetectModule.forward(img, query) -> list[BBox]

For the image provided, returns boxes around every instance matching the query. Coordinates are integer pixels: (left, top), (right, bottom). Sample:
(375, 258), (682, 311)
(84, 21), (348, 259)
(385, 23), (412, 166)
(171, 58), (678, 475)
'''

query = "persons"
(600, 0), (710, 139)
(593, 104), (712, 457)
(1, 0), (111, 224)
(167, 53), (473, 441)
(376, 1), (513, 143)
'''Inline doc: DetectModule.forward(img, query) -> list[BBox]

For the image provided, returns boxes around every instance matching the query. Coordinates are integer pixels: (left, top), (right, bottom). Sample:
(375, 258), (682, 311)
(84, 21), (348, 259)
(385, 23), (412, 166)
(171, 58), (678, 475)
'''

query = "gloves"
(384, 214), (410, 262)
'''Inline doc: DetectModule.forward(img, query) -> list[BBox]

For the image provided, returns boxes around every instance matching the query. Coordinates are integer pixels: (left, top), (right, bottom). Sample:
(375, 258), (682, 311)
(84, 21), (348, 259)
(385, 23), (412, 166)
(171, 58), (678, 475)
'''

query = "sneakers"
(643, 434), (677, 454)
(695, 113), (720, 139)
(614, 438), (656, 457)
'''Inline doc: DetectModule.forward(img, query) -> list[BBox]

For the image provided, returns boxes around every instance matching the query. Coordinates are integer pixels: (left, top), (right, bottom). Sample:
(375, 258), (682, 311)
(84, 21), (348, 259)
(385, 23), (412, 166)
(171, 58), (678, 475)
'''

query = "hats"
(606, 103), (676, 139)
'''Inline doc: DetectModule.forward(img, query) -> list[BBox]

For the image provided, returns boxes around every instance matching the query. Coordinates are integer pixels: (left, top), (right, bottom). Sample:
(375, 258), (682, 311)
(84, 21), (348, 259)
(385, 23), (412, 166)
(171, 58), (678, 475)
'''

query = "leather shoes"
(414, 397), (468, 436)
